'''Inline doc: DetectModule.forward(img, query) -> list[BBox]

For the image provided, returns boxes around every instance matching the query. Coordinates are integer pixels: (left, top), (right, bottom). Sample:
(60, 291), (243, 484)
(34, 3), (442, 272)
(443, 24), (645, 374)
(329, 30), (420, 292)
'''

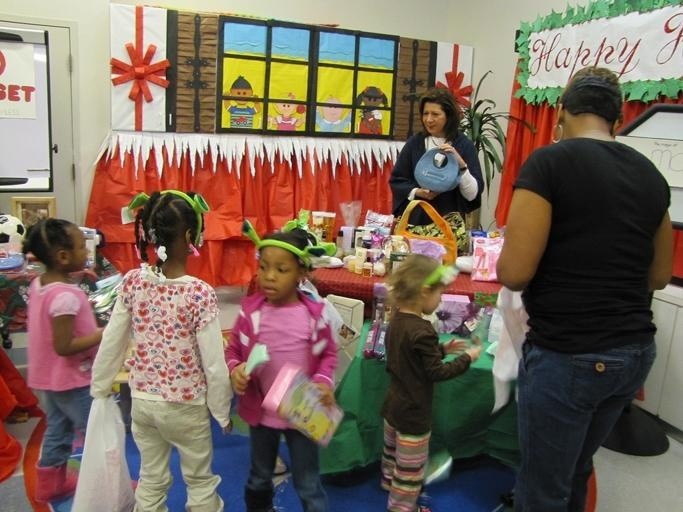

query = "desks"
(255, 255), (507, 328)
(319, 333), (521, 484)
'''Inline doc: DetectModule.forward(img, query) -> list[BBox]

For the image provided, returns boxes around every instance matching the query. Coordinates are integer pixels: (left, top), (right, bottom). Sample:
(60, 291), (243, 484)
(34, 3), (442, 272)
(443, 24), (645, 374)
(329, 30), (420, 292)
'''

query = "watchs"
(459, 165), (469, 173)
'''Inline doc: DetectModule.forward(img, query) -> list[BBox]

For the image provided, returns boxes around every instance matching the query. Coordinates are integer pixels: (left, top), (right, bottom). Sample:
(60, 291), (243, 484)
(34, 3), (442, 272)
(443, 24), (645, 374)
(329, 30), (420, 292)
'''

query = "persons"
(82, 188), (233, 511)
(491, 66), (674, 510)
(19, 216), (105, 509)
(218, 225), (344, 511)
(386, 85), (484, 232)
(375, 256), (482, 511)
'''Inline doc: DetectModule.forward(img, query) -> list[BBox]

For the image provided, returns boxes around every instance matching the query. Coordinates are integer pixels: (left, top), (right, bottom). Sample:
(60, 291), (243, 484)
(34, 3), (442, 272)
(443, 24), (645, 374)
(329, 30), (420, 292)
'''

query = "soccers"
(1, 214), (26, 251)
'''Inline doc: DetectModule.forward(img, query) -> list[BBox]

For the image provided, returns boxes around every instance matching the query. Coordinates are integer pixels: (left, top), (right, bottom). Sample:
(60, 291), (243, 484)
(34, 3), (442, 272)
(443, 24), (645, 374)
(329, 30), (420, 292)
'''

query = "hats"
(563, 65), (622, 95)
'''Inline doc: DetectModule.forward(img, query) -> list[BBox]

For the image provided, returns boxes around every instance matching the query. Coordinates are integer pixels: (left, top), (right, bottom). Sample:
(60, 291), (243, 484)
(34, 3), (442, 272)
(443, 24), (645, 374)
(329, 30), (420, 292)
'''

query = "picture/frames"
(215, 13), (401, 140)
(7, 194), (57, 251)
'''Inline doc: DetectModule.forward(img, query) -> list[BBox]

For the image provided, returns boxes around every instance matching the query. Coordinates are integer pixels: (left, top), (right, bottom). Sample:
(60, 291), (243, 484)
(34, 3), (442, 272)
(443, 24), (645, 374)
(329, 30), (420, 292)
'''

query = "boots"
(33, 459), (79, 503)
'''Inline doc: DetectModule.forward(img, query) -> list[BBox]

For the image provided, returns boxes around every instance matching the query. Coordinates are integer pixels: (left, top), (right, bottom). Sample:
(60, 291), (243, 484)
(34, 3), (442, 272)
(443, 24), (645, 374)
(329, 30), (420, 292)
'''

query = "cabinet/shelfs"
(629, 285), (683, 435)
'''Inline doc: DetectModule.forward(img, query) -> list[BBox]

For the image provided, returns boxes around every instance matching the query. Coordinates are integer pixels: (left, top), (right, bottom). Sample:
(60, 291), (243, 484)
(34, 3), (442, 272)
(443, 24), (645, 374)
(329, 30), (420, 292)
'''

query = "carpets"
(17, 324), (599, 512)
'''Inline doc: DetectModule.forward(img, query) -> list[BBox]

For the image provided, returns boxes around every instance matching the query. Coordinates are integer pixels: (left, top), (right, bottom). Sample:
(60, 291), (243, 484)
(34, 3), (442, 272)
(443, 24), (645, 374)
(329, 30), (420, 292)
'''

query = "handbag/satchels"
(394, 199), (457, 268)
(414, 147), (459, 193)
(470, 220), (504, 283)
